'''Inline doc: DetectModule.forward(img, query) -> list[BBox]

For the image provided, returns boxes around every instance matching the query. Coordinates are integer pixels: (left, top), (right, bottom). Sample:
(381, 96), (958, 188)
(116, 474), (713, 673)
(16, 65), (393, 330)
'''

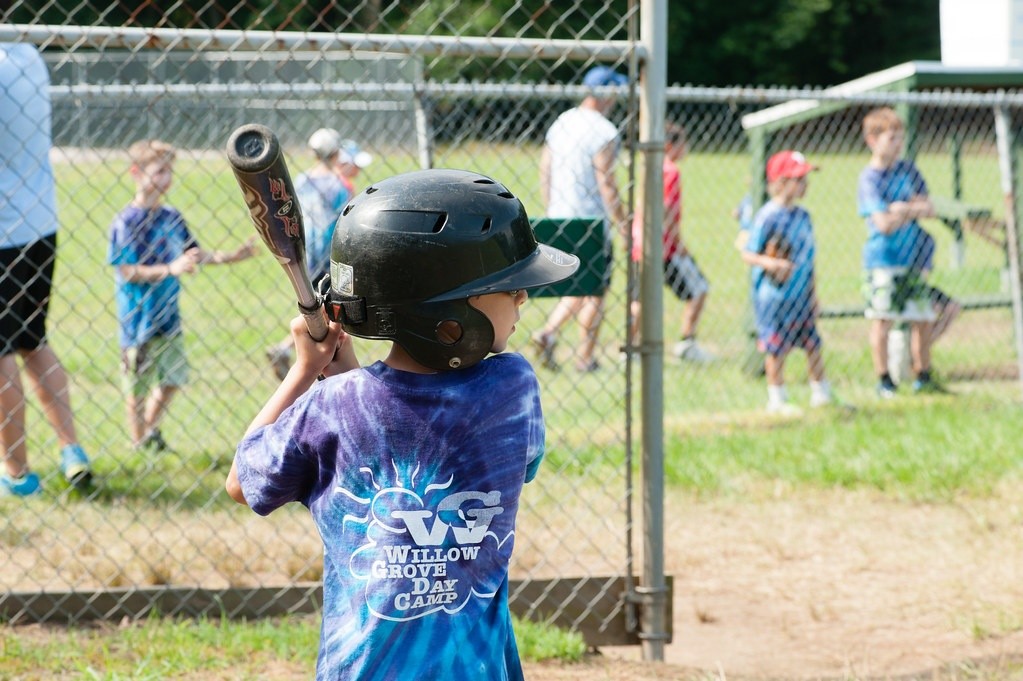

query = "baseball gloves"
(764, 235), (791, 289)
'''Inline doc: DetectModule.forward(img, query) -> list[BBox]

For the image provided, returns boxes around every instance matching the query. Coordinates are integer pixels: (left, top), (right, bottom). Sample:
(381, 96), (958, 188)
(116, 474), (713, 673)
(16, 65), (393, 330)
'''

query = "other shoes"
(574, 355), (600, 375)
(881, 387), (902, 400)
(2, 472), (42, 499)
(768, 406), (807, 425)
(619, 345), (642, 362)
(145, 429), (165, 451)
(528, 332), (564, 374)
(915, 378), (952, 395)
(59, 445), (94, 491)
(814, 398), (856, 421)
(676, 340), (711, 365)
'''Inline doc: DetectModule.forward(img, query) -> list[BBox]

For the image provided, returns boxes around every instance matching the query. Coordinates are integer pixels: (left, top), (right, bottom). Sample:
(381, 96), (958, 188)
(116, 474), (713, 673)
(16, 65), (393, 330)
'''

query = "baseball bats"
(225, 119), (346, 367)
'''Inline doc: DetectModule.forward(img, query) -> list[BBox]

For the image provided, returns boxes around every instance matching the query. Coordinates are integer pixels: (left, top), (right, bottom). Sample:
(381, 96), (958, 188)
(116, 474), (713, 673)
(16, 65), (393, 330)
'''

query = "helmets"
(318, 169), (579, 376)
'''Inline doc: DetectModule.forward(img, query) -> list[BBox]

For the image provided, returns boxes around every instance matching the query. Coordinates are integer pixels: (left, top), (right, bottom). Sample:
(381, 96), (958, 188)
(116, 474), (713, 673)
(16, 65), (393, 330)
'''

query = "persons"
(226, 169), (580, 681)
(0, 41), (94, 498)
(631, 122), (714, 363)
(857, 107), (946, 398)
(107, 138), (259, 451)
(532, 65), (630, 373)
(293, 127), (372, 276)
(741, 151), (836, 416)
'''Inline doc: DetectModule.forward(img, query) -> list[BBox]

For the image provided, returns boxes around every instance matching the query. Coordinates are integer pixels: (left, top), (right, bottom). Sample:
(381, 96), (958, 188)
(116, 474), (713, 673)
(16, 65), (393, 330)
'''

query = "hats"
(308, 129), (342, 155)
(768, 151), (820, 178)
(582, 67), (628, 88)
(335, 140), (374, 168)
